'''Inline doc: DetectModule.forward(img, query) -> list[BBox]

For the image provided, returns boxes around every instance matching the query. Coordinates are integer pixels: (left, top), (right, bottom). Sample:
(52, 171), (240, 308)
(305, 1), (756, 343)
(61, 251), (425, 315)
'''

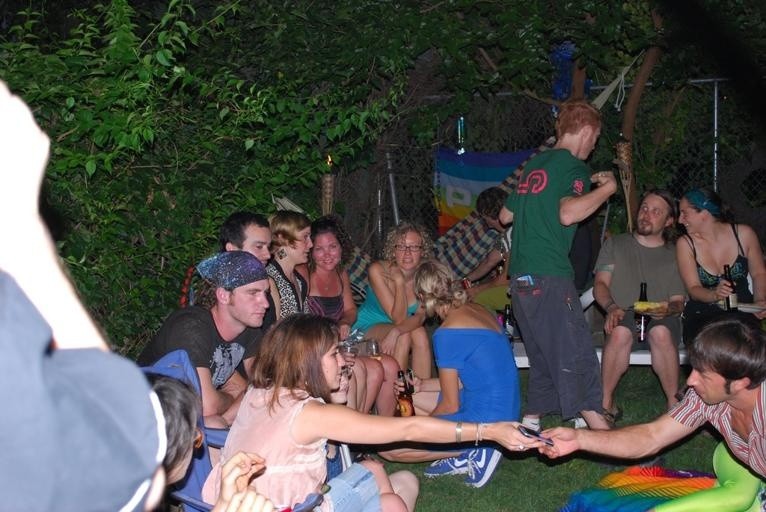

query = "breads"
(632, 302), (666, 312)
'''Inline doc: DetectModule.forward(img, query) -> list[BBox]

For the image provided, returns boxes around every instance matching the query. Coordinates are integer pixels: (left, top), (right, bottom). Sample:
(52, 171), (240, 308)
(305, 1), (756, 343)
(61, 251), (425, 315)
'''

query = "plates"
(738, 303), (766, 314)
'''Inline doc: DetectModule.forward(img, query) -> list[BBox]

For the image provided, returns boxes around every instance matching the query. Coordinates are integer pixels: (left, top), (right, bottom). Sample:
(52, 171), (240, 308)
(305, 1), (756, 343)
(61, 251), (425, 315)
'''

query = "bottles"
(724, 264), (738, 314)
(366, 338), (379, 355)
(496, 249), (504, 276)
(503, 304), (515, 342)
(405, 369), (414, 393)
(398, 371), (415, 417)
(633, 282), (652, 343)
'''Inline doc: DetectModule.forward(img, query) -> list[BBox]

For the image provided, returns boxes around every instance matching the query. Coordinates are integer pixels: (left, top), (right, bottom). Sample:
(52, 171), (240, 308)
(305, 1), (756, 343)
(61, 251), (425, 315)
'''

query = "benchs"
(511, 341), (692, 371)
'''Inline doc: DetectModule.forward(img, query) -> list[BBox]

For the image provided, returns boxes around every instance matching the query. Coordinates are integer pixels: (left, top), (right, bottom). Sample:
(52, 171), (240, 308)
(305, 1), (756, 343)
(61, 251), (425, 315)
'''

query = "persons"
(537, 311), (766, 480)
(676, 186), (765, 345)
(594, 186), (689, 420)
(137, 186), (522, 512)
(0, 77), (168, 512)
(498, 101), (617, 433)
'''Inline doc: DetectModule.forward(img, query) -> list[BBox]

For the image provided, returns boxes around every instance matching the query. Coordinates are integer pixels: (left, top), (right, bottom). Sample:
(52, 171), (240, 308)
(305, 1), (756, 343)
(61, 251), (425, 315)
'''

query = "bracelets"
(713, 294), (718, 301)
(606, 301), (615, 310)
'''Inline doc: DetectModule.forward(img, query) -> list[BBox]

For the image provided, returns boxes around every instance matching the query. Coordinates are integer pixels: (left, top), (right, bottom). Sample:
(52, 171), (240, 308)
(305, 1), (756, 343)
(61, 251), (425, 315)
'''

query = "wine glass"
(343, 340), (361, 376)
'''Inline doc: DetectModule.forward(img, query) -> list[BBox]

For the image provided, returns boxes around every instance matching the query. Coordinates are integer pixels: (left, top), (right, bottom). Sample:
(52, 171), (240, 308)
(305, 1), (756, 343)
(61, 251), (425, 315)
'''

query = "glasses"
(193, 428), (203, 448)
(395, 245), (422, 251)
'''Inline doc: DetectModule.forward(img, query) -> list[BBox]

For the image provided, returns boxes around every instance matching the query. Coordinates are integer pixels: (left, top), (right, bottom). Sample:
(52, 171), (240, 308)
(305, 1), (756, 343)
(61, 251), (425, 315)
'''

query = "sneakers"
(424, 453), (467, 478)
(465, 447), (502, 489)
(601, 405), (621, 428)
